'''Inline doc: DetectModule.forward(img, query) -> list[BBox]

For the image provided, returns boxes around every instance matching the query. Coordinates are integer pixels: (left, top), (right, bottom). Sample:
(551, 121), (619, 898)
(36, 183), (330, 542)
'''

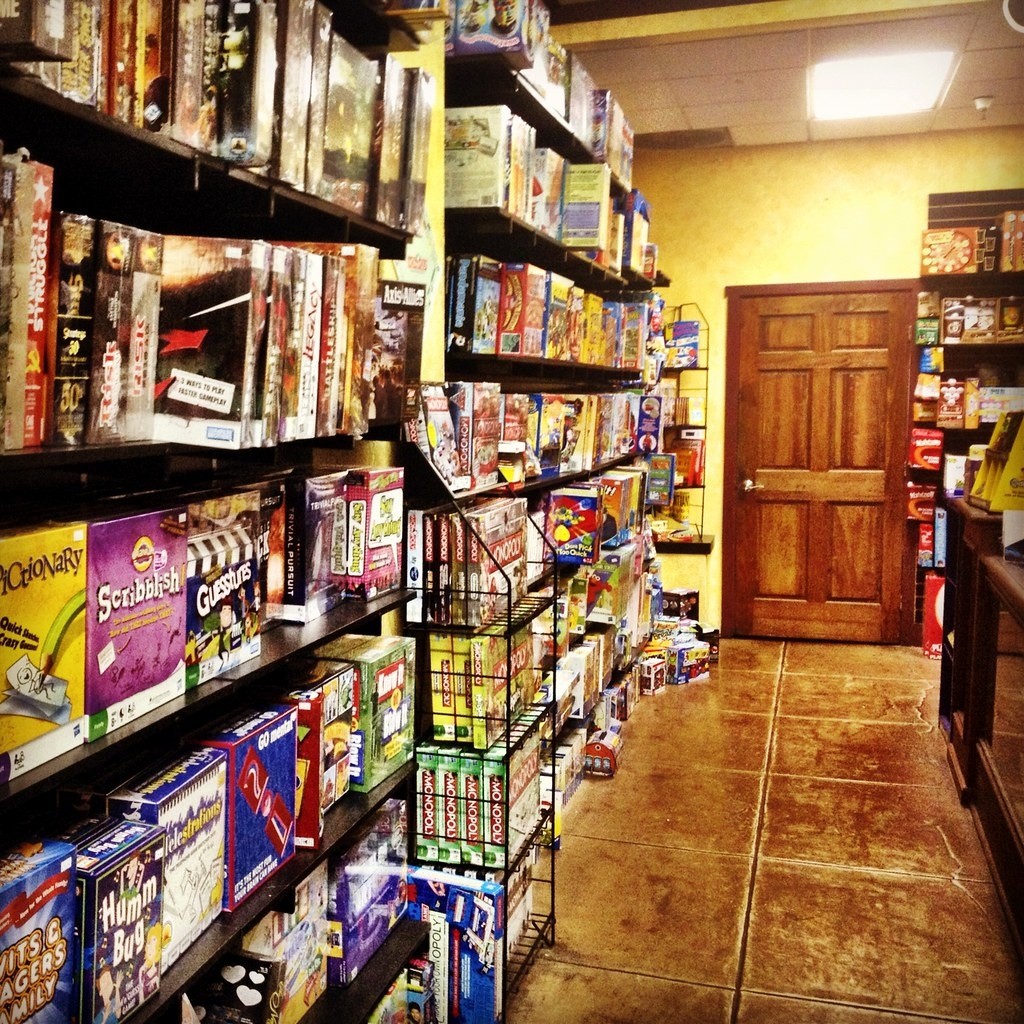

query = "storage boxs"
(906, 208), (1024, 657)
(0, 0), (724, 1024)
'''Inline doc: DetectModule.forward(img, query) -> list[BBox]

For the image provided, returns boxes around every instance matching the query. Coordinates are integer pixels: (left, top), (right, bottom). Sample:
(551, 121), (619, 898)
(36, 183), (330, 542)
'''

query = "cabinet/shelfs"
(919, 272), (1024, 440)
(0, 0), (449, 1024)
(405, 440), (557, 1011)
(936, 497), (1023, 953)
(661, 304), (710, 538)
(446, 50), (646, 786)
